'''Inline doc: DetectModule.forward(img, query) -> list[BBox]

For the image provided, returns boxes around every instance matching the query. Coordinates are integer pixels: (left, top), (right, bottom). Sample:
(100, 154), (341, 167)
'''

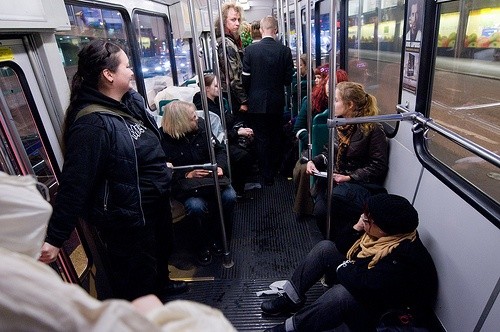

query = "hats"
(302, 54), (308, 65)
(315, 64), (329, 75)
(368, 194), (418, 236)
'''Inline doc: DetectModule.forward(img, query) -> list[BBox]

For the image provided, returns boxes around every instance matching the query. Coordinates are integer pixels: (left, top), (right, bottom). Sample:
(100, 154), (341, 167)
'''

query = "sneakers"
(264, 323), (286, 332)
(261, 292), (307, 316)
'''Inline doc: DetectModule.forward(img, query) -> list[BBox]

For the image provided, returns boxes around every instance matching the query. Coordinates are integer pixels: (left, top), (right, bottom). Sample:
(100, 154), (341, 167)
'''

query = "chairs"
(287, 68), (340, 204)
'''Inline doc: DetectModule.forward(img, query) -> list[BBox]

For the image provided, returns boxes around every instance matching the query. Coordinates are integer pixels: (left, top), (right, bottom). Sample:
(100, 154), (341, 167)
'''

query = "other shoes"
(172, 281), (188, 294)
(265, 177), (273, 186)
(196, 249), (212, 265)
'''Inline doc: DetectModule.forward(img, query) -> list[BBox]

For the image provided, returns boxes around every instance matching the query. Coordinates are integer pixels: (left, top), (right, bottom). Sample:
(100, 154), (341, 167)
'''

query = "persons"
(239, 20), (262, 48)
(260, 193), (439, 332)
(285, 56), (348, 220)
(306, 81), (391, 245)
(155, 99), (236, 264)
(193, 73), (254, 184)
(243, 14), (295, 183)
(213, 3), (249, 136)
(405, 2), (421, 41)
(38, 37), (178, 305)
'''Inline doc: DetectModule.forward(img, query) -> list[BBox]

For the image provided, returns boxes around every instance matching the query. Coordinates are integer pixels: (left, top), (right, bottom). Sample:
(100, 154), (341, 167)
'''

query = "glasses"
(363, 217), (375, 226)
(100, 40), (120, 63)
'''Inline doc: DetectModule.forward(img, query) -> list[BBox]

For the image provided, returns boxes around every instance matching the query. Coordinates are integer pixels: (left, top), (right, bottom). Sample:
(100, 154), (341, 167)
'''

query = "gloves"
(297, 129), (309, 149)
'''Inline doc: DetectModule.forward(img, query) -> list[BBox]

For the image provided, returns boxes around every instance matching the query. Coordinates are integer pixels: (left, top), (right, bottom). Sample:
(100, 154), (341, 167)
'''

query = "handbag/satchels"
(172, 167), (231, 196)
(230, 142), (250, 170)
